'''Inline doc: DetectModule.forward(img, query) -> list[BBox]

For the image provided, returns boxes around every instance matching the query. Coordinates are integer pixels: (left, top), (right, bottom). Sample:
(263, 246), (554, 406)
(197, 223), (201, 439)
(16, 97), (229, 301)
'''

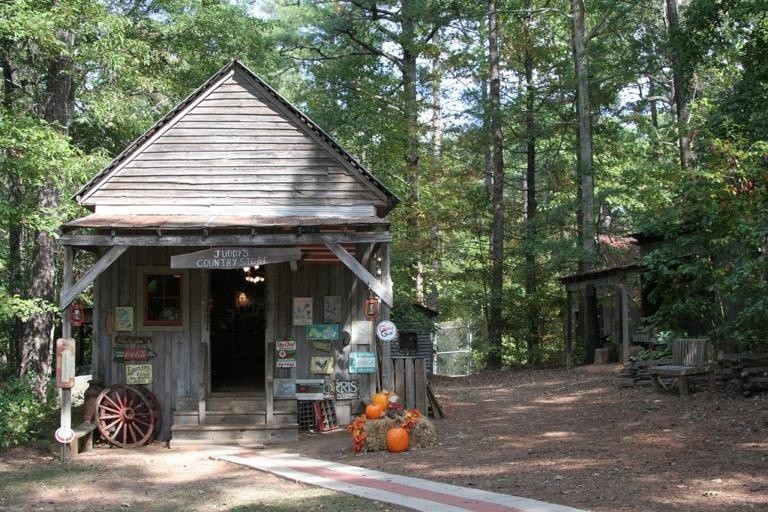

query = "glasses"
(245, 266), (266, 285)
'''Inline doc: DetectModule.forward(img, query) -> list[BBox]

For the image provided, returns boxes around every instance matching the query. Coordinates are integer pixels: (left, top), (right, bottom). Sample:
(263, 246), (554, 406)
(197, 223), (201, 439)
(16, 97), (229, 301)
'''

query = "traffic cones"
(374, 394), (388, 410)
(367, 404), (383, 419)
(387, 428), (409, 452)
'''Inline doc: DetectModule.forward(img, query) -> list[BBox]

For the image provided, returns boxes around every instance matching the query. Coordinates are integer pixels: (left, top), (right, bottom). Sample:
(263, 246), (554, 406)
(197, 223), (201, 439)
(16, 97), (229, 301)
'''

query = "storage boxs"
(649, 338), (719, 396)
